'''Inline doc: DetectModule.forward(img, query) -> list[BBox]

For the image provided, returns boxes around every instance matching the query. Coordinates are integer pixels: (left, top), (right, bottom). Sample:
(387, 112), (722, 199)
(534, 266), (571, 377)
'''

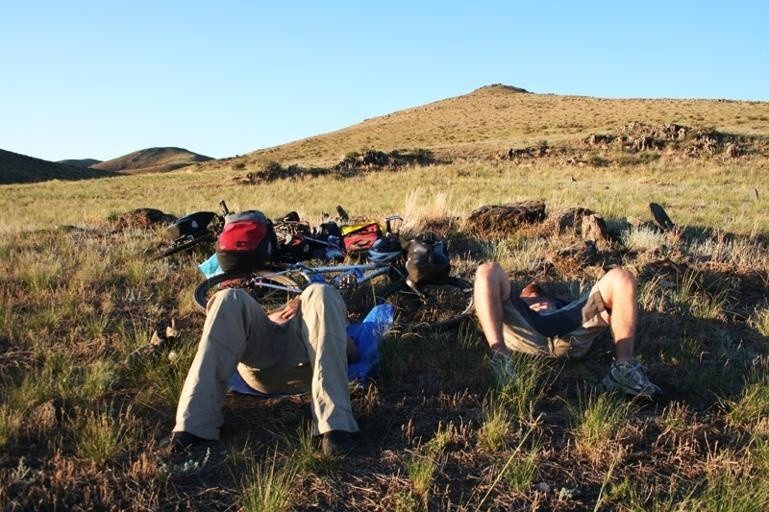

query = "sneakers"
(488, 346), (515, 382)
(323, 430), (352, 458)
(170, 432), (199, 461)
(602, 359), (663, 397)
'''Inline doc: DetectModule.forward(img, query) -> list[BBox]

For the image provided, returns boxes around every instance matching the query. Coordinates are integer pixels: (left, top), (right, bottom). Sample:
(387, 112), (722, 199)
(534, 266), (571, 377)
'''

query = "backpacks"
(216, 208), (276, 271)
(408, 236), (451, 285)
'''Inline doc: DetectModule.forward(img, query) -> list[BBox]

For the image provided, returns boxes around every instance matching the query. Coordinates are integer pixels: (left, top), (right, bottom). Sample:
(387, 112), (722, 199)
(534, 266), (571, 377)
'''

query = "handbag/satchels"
(342, 222), (381, 253)
(167, 212), (216, 239)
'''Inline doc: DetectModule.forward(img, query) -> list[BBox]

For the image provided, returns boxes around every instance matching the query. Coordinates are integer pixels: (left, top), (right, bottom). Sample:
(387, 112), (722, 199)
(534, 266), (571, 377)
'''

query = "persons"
(153, 282), (359, 469)
(472, 261), (665, 401)
